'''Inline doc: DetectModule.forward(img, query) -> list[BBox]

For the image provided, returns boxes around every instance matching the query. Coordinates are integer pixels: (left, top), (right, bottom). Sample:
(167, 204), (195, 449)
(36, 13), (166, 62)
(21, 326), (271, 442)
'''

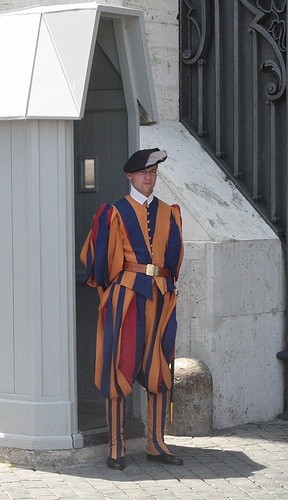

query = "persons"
(79, 148), (183, 470)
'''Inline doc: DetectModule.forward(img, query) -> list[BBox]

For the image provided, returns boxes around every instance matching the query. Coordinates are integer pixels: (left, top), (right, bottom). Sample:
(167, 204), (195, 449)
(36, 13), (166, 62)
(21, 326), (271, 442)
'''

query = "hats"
(123, 147), (168, 173)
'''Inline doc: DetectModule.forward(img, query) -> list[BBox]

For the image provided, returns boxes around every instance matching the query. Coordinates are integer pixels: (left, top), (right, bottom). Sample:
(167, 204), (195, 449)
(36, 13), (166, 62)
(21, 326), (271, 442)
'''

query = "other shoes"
(107, 456), (127, 470)
(146, 453), (184, 465)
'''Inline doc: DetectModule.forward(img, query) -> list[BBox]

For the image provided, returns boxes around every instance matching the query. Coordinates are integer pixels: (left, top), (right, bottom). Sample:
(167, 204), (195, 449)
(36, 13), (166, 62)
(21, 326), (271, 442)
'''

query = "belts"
(123, 261), (171, 278)
